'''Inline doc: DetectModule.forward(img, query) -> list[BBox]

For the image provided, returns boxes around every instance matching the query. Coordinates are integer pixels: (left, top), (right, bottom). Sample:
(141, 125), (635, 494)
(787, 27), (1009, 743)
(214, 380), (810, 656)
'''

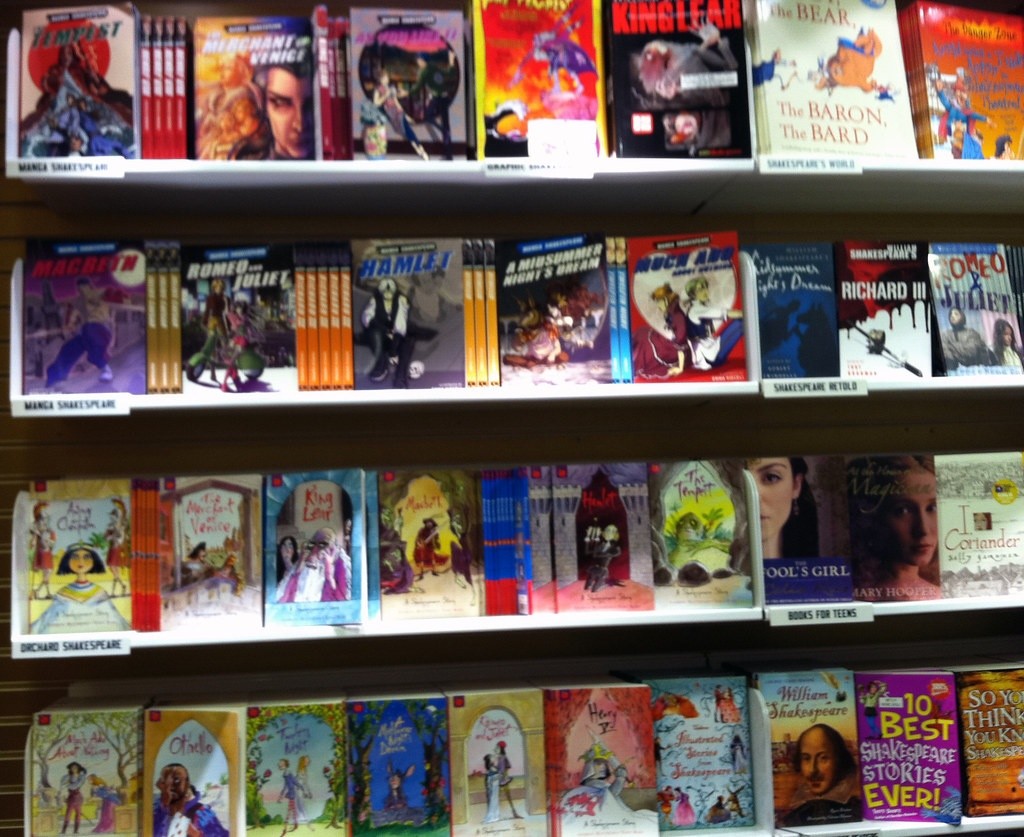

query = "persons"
(846, 453), (944, 603)
(744, 453), (819, 566)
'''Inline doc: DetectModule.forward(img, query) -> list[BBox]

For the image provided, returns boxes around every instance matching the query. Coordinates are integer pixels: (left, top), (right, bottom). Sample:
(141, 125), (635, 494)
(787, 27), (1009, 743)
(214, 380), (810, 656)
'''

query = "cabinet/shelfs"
(0, 0), (1024, 836)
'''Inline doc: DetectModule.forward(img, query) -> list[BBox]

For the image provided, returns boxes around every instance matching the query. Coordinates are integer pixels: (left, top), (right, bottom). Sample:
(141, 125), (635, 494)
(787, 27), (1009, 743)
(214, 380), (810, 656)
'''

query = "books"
(11, 0), (1024, 837)
